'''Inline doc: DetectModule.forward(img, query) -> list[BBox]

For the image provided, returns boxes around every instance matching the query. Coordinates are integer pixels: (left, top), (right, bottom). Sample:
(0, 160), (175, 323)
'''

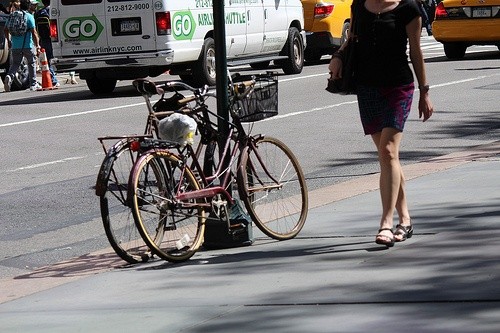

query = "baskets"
(228, 75), (280, 122)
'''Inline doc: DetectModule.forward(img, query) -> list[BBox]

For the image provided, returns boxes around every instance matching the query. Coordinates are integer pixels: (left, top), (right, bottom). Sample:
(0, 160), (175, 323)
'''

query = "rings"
(329, 72), (333, 74)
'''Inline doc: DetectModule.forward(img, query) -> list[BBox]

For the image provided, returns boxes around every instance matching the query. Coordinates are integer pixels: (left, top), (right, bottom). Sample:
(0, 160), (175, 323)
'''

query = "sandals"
(394, 217), (413, 242)
(376, 228), (394, 247)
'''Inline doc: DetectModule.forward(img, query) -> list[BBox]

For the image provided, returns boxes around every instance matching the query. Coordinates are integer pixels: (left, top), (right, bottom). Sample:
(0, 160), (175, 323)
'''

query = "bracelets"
(331, 51), (342, 58)
(419, 85), (429, 90)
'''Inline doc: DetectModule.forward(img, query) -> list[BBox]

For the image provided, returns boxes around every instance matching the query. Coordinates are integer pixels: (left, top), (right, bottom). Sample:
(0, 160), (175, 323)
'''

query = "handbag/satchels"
(325, 4), (356, 95)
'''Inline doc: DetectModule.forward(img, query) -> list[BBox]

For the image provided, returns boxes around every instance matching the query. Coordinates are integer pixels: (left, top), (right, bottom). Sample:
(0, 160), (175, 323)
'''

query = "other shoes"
(4, 75), (11, 92)
(30, 82), (38, 90)
(71, 79), (79, 85)
(53, 82), (60, 87)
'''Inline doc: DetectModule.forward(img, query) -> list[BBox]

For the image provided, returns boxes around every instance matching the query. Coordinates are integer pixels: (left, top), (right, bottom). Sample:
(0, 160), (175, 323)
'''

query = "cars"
(433, 0), (500, 60)
(301, 0), (354, 63)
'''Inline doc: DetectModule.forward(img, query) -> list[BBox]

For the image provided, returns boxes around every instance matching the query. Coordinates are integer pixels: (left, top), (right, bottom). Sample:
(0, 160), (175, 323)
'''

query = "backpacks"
(36, 17), (50, 40)
(7, 9), (31, 36)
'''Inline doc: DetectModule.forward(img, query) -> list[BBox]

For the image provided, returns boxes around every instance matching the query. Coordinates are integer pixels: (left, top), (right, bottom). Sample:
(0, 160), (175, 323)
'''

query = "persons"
(328, 0), (442, 248)
(0, 0), (78, 91)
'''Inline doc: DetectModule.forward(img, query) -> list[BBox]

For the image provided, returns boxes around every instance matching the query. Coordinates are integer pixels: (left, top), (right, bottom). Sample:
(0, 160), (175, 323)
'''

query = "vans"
(48, 0), (307, 97)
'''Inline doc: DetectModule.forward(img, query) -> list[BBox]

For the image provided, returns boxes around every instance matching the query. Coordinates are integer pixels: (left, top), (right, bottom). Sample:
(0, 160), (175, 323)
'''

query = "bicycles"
(89, 70), (308, 264)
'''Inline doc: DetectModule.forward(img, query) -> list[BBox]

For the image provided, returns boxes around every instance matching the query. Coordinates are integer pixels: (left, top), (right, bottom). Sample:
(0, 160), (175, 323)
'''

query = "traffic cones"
(36, 48), (58, 91)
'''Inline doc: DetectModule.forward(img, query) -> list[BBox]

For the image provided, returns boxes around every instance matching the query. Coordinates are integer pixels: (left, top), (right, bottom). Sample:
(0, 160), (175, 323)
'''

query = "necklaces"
(375, 0), (386, 15)
